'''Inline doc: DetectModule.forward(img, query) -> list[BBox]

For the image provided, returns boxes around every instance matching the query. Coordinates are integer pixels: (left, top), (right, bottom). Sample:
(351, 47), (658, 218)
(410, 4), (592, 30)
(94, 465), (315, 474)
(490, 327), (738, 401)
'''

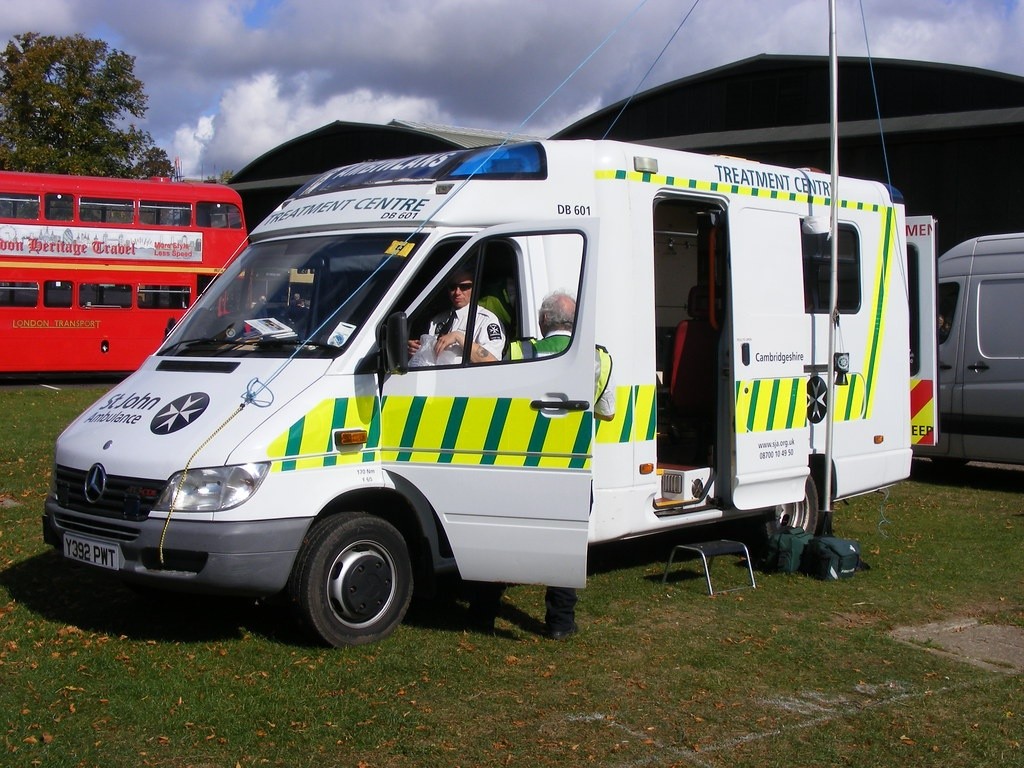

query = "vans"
(910, 232), (1024, 475)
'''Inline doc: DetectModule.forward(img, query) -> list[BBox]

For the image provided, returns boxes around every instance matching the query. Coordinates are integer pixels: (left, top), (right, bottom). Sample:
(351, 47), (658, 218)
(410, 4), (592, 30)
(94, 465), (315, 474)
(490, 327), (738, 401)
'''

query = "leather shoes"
(550, 620), (579, 638)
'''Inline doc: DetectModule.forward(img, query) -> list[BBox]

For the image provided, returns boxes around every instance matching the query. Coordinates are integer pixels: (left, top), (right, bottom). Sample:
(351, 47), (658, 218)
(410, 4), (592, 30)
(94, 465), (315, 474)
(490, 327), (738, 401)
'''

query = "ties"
(437, 311), (458, 341)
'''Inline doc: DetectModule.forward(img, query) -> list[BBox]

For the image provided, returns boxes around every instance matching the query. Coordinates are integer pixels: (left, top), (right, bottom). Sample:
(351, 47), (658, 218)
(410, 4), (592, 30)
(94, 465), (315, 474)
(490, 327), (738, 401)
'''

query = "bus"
(0, 171), (250, 385)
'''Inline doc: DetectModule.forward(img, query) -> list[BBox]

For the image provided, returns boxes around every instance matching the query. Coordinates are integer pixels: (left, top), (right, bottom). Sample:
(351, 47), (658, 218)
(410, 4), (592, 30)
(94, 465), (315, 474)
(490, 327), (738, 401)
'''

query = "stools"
(663, 538), (756, 597)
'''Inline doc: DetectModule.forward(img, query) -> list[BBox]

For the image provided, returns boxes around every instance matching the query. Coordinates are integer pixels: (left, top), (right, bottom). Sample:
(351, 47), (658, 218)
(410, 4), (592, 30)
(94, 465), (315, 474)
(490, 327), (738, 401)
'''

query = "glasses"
(447, 283), (473, 291)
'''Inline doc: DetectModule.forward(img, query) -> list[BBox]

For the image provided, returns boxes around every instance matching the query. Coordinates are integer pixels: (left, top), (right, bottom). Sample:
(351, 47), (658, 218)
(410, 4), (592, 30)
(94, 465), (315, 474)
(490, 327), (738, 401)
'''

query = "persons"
(291, 293), (310, 309)
(406, 269), (505, 367)
(254, 295), (266, 317)
(464, 293), (616, 638)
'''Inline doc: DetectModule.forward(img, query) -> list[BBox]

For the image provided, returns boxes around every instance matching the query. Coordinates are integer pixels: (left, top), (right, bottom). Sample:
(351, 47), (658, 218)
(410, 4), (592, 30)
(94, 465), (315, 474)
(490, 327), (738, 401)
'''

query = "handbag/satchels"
(751, 526), (859, 581)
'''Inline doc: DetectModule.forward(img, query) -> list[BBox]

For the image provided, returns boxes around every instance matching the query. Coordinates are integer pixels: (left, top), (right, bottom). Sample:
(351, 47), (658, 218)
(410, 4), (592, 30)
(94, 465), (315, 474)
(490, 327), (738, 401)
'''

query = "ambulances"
(41, 137), (943, 652)
